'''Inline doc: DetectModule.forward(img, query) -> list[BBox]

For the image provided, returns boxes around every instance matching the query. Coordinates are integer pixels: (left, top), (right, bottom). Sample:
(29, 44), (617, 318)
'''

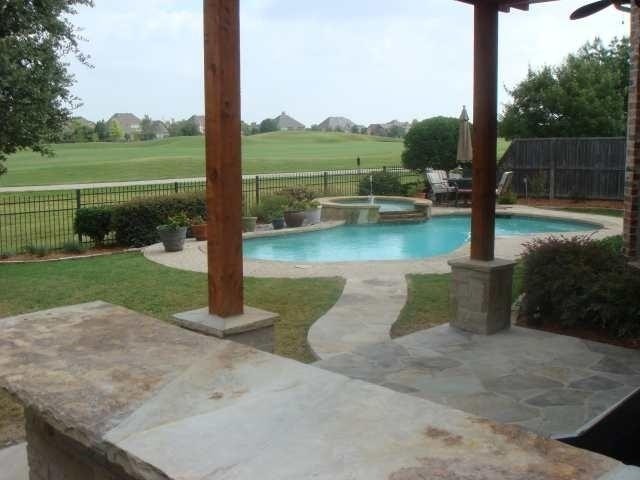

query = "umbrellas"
(456, 105), (472, 168)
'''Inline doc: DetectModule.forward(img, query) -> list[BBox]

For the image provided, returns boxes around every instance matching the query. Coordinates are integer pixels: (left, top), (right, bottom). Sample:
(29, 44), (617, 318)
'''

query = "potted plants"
(416, 183), (427, 198)
(284, 203), (304, 226)
(184, 215), (207, 240)
(304, 200), (322, 224)
(155, 215), (188, 251)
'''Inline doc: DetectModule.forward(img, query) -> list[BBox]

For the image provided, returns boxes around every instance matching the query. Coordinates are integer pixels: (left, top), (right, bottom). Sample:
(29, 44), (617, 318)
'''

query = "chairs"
(425, 170), (513, 207)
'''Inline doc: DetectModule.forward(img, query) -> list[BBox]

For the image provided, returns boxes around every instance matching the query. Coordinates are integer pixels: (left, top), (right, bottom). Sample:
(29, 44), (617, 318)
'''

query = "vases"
(242, 216), (257, 232)
(272, 219), (284, 229)
